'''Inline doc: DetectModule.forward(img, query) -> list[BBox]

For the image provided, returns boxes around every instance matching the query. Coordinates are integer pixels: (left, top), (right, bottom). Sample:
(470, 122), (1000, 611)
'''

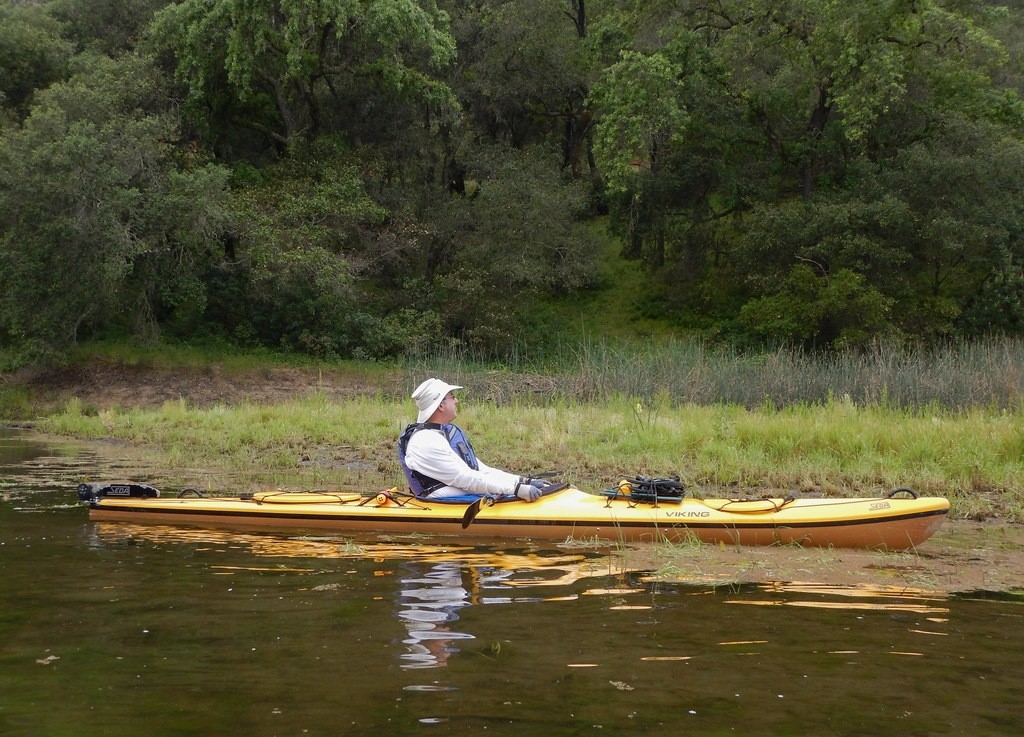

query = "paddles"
(460, 492), (502, 530)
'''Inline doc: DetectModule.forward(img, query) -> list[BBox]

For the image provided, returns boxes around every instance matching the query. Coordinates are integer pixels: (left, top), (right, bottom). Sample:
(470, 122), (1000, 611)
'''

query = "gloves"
(514, 476), (551, 503)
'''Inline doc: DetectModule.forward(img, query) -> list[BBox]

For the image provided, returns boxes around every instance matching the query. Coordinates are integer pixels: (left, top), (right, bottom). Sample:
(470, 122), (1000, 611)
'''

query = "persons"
(399, 377), (551, 502)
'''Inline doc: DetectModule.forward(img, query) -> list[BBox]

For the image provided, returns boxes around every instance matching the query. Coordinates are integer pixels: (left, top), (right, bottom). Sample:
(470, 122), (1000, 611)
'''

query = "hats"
(411, 377), (463, 424)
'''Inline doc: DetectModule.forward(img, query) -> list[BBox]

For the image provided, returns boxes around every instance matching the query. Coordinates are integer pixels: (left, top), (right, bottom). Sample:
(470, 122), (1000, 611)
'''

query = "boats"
(77, 476), (951, 550)
